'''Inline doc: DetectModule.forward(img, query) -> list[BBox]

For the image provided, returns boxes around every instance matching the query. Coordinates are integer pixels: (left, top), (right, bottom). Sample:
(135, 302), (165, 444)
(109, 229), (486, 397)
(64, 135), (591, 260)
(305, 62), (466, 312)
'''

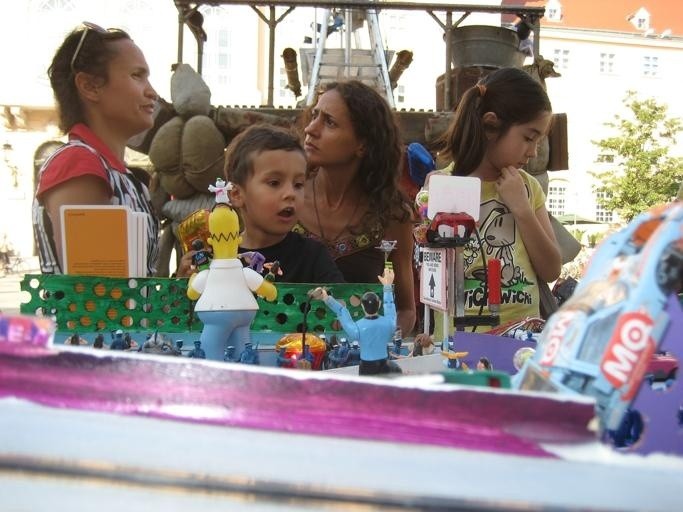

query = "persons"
(80, 327), (512, 370)
(188, 177), (287, 364)
(33, 21), (160, 277)
(176, 123), (347, 284)
(292, 80), (416, 338)
(312, 267), (398, 376)
(80, 327), (512, 408)
(424, 67), (562, 342)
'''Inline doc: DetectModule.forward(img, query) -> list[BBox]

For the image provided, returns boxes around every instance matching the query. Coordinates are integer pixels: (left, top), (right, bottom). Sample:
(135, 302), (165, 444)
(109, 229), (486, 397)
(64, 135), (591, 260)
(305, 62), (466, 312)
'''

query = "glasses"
(70, 21), (106, 76)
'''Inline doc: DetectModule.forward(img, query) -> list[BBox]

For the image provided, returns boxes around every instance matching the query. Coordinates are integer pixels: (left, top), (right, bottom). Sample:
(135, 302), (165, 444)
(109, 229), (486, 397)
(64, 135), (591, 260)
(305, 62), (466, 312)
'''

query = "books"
(61, 204), (148, 277)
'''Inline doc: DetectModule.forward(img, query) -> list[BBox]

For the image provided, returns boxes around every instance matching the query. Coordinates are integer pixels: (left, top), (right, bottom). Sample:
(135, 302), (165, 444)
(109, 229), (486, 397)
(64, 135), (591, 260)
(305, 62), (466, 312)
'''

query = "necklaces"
(312, 174), (365, 242)
